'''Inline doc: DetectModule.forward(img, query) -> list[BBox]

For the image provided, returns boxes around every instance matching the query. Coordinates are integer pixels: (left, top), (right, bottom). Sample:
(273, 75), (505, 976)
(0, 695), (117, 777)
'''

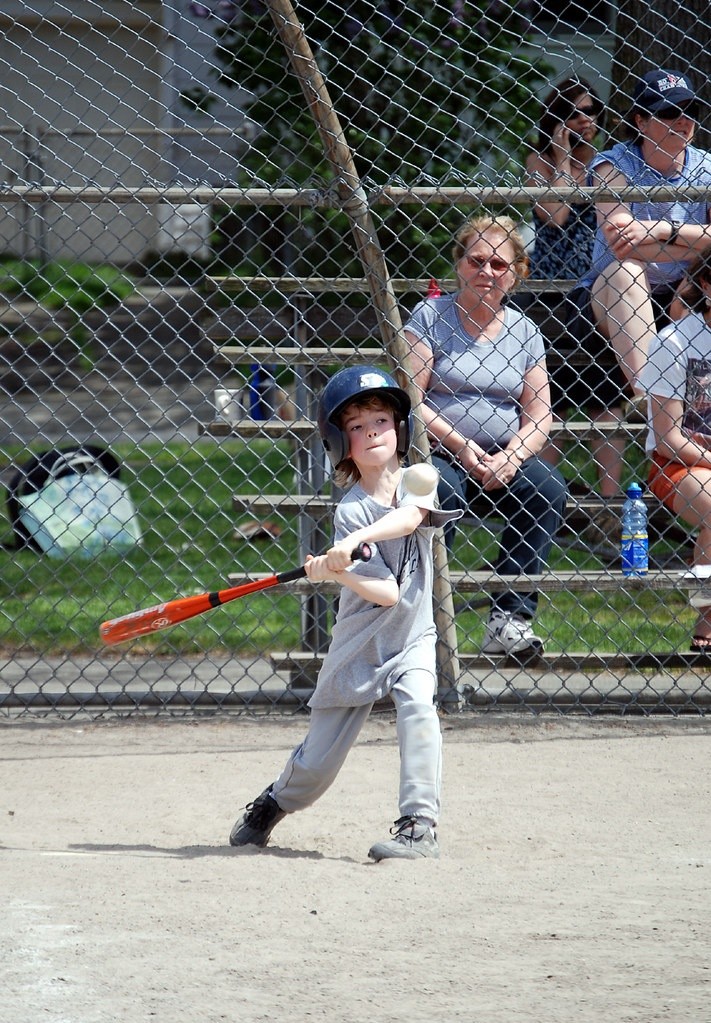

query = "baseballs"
(405, 463), (436, 496)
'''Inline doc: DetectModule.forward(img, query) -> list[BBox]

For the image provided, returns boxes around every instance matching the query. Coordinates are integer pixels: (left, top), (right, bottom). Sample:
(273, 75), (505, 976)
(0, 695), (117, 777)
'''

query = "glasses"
(465, 256), (514, 271)
(637, 103), (699, 120)
(567, 106), (596, 120)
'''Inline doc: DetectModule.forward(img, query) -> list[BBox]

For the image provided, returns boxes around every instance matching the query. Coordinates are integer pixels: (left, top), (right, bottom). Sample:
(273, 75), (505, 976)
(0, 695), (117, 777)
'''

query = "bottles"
(621, 483), (648, 576)
(250, 364), (277, 420)
(428, 278), (441, 300)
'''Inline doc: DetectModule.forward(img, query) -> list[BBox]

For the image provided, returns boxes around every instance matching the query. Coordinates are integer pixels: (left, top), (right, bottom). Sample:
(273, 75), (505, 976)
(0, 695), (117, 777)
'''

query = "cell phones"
(569, 134), (585, 148)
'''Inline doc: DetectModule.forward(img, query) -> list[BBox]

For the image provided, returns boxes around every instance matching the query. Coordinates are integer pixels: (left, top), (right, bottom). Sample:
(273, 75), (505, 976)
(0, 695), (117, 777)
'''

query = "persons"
(229, 366), (464, 864)
(506, 75), (635, 498)
(636, 243), (711, 653)
(568, 68), (711, 418)
(399, 214), (567, 664)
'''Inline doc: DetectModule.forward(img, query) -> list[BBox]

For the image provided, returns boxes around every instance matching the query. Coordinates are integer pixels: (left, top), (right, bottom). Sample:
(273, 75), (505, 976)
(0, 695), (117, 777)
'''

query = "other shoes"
(625, 395), (648, 454)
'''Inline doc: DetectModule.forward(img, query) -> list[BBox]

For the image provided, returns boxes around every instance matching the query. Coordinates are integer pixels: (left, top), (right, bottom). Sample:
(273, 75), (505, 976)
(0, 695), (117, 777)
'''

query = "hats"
(630, 70), (707, 110)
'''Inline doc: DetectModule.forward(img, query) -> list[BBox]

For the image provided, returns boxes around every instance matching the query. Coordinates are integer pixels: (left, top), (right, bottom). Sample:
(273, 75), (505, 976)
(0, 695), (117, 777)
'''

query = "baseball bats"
(98, 542), (372, 649)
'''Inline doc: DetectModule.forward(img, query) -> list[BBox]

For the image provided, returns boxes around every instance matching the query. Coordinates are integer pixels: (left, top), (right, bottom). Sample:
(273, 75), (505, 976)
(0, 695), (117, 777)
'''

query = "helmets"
(316, 365), (414, 472)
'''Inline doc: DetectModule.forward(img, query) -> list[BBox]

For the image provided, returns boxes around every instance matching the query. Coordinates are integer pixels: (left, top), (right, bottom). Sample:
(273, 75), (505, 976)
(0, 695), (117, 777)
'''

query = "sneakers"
(368, 815), (440, 861)
(229, 783), (287, 848)
(690, 635), (711, 652)
(482, 611), (545, 657)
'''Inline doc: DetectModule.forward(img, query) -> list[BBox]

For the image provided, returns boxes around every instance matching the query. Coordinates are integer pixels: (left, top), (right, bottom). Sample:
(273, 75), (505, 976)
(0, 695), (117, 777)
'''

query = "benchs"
(199, 275), (711, 686)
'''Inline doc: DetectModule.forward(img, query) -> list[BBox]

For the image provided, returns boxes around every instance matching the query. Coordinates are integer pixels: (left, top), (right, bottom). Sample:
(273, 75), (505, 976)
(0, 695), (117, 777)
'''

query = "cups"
(213, 389), (244, 422)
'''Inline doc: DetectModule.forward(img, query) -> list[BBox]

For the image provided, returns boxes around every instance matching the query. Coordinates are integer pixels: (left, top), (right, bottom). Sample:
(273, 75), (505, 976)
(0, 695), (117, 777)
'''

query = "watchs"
(505, 445), (524, 463)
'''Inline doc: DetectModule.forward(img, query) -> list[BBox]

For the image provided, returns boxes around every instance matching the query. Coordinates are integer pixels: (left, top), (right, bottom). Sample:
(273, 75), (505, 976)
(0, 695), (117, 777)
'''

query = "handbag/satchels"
(6, 444), (145, 559)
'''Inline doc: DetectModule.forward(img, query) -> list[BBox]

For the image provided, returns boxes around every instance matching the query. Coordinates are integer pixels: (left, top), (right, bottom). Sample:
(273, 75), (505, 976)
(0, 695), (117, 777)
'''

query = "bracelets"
(667, 220), (680, 246)
